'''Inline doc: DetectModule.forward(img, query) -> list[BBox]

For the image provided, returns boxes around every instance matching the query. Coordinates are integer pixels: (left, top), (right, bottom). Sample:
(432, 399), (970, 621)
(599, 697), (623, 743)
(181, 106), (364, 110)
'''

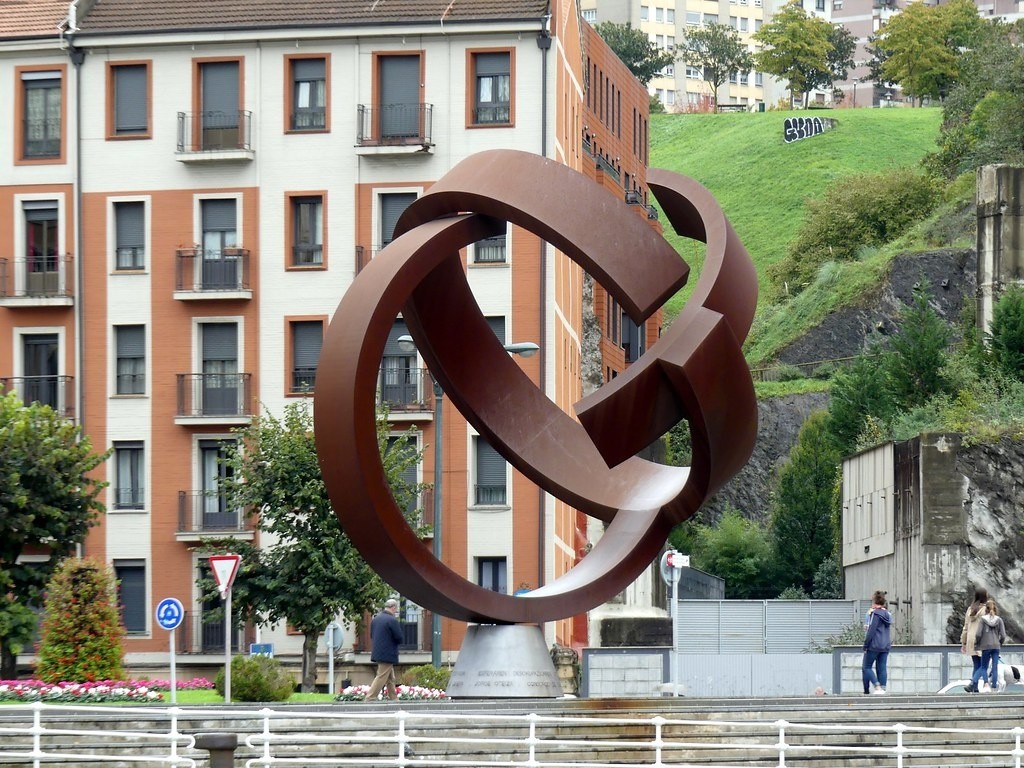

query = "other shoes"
(983, 683), (991, 693)
(874, 690), (886, 694)
(992, 688), (997, 694)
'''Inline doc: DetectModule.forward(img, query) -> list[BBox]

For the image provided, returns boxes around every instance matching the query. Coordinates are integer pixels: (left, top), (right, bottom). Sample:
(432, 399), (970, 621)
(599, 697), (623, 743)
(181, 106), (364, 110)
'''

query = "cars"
(936, 681), (1024, 695)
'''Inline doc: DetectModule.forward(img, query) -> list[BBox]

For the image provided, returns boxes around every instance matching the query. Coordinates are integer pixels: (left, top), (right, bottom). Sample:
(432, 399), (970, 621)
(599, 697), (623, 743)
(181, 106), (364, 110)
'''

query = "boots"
(964, 679), (977, 692)
(974, 684), (979, 693)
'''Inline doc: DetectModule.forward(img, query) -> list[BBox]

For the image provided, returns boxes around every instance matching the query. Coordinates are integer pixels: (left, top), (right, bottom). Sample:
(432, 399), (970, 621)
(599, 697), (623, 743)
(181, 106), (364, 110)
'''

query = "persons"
(861, 595), (891, 695)
(861, 590), (888, 695)
(364, 598), (404, 702)
(960, 588), (993, 693)
(974, 601), (1007, 694)
